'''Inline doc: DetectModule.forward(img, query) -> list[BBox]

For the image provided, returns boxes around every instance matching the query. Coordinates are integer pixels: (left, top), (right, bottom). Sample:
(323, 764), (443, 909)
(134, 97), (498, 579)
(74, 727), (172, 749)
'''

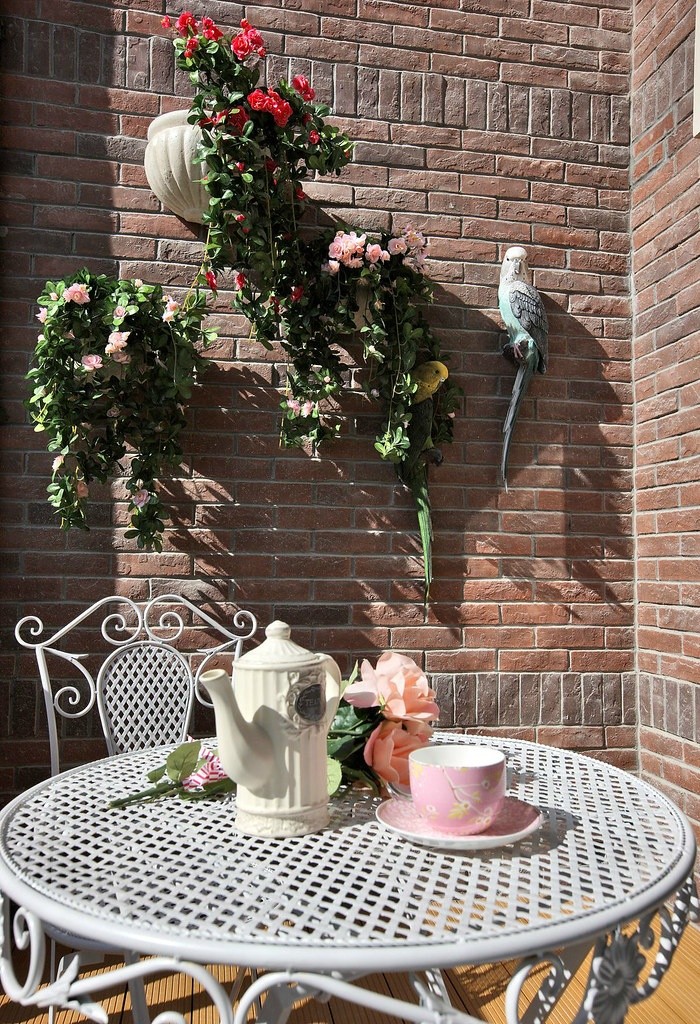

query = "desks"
(1, 727), (700, 1024)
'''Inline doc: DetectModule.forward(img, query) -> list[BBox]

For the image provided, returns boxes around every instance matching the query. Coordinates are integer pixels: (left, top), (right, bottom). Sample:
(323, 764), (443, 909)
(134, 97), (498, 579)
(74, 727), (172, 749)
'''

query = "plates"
(376, 795), (544, 851)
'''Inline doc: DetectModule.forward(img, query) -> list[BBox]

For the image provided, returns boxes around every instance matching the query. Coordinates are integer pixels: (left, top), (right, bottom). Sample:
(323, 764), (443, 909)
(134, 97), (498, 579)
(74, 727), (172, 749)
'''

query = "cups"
(408, 744), (507, 836)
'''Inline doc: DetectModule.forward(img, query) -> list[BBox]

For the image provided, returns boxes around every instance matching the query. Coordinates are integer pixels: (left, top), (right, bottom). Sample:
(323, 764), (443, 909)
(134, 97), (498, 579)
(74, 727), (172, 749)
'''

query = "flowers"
(108, 652), (438, 804)
(24, 267), (222, 556)
(305, 222), (438, 465)
(161, 12), (355, 353)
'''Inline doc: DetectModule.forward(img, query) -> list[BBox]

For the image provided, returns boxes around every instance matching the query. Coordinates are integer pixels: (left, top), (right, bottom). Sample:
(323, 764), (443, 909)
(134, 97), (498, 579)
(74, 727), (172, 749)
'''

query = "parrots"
(490, 244), (548, 495)
(393, 359), (449, 587)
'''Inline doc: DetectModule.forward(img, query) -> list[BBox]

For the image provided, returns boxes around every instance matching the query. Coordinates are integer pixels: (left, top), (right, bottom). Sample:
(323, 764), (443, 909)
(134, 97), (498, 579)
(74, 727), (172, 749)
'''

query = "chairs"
(14, 595), (258, 1024)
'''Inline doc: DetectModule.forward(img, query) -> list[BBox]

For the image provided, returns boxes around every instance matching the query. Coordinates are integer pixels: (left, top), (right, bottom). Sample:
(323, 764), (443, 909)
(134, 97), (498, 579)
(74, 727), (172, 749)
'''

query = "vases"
(143, 108), (227, 227)
(317, 282), (375, 335)
(97, 354), (145, 396)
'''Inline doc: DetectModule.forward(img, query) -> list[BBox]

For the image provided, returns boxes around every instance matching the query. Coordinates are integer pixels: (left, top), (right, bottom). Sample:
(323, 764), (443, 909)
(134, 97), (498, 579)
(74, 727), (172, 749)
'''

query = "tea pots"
(199, 620), (342, 838)
